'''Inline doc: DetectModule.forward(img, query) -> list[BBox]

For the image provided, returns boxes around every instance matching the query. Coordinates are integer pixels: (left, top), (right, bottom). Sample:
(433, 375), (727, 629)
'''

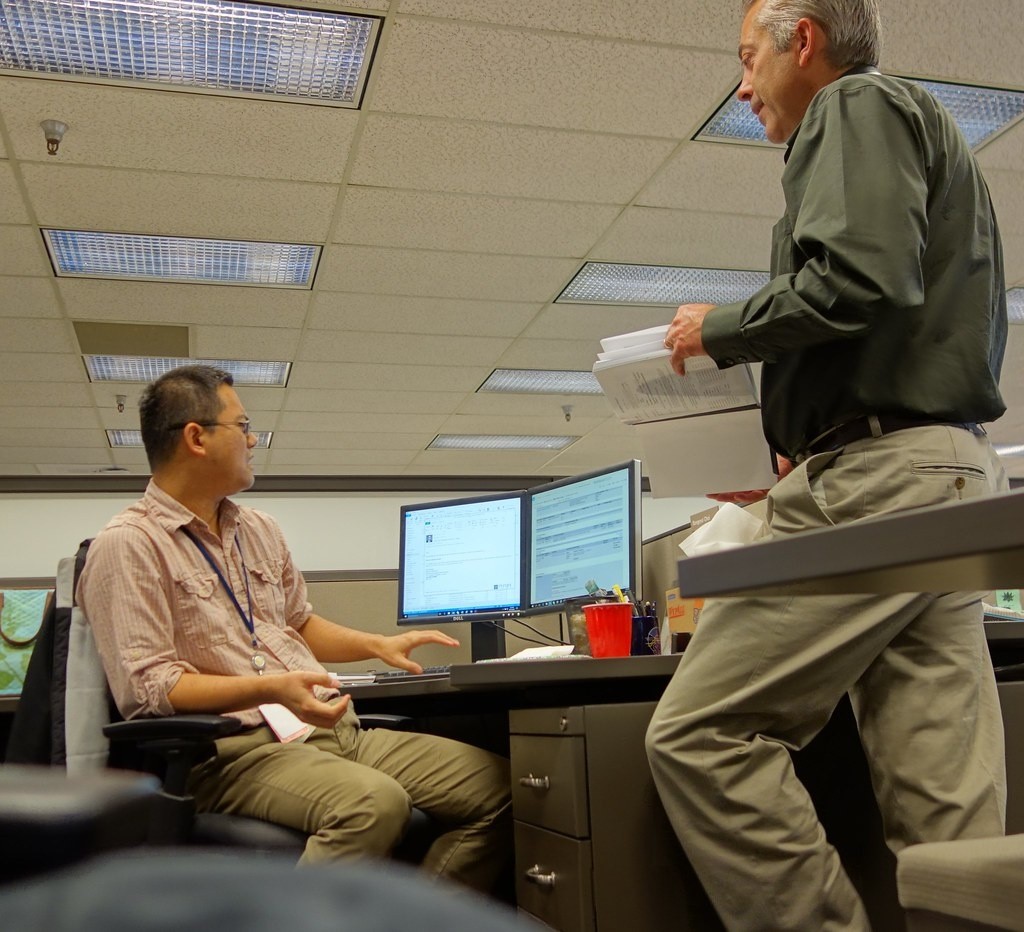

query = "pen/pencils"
(612, 579), (660, 619)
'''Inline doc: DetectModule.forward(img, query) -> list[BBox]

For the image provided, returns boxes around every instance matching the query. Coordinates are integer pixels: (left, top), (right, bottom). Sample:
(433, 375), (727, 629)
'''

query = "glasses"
(164, 416), (252, 436)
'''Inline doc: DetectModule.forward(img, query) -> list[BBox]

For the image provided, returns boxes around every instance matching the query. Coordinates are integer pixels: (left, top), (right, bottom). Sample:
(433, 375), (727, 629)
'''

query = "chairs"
(20, 538), (440, 860)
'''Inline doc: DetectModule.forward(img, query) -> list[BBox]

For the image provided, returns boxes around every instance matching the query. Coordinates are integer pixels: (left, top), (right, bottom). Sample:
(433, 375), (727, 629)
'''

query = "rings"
(663, 339), (671, 348)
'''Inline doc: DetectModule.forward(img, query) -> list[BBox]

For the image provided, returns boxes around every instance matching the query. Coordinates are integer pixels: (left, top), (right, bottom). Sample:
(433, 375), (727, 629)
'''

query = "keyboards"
(477, 655), (593, 660)
(378, 665), (450, 683)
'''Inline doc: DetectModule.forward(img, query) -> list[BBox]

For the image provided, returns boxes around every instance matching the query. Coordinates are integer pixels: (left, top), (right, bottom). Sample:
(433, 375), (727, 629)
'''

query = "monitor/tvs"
(525, 459), (642, 656)
(397, 489), (527, 663)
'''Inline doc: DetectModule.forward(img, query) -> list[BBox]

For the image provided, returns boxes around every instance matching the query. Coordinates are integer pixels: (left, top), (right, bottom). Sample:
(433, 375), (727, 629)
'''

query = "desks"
(300, 497), (1024, 932)
(678, 485), (1024, 932)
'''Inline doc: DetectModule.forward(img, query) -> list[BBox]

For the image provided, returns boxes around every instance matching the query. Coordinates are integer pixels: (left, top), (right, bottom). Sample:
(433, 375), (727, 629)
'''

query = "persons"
(642, 0), (1010, 932)
(73, 365), (512, 890)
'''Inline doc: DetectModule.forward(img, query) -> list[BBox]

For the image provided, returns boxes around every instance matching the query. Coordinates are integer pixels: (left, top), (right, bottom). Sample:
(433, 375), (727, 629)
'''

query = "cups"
(582, 603), (633, 658)
(633, 616), (660, 655)
(565, 597), (618, 656)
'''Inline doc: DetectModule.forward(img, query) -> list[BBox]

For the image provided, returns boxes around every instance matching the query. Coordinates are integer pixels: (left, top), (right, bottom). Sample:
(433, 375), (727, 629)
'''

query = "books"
(592, 324), (761, 426)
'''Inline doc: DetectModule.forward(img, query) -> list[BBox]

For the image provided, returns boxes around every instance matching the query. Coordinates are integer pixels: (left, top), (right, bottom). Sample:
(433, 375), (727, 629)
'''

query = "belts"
(794, 407), (985, 467)
